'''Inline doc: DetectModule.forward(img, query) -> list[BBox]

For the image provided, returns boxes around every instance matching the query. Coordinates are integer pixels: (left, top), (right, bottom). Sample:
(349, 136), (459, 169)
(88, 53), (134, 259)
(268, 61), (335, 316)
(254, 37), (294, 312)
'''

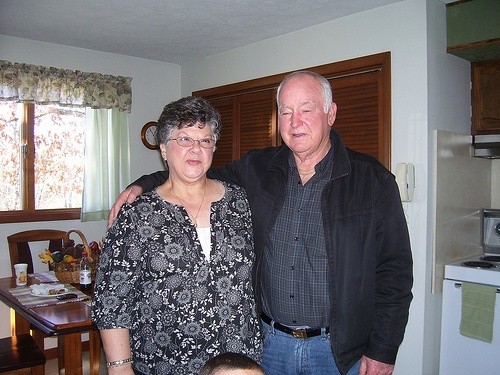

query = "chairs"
(0, 334), (46, 375)
(7, 230), (69, 375)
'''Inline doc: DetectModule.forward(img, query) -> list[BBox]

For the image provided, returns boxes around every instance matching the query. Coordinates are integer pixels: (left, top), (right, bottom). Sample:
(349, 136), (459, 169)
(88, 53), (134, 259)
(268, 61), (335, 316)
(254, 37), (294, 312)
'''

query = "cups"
(14, 264), (28, 285)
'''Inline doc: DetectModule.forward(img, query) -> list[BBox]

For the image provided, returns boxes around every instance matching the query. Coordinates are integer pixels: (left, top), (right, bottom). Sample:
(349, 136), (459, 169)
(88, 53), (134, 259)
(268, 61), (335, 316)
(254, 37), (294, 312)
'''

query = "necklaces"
(169, 183), (206, 228)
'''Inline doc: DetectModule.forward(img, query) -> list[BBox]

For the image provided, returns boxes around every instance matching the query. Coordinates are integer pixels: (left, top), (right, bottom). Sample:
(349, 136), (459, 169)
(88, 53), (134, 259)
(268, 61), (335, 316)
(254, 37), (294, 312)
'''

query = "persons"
(91, 97), (262, 375)
(199, 352), (265, 375)
(107, 70), (413, 375)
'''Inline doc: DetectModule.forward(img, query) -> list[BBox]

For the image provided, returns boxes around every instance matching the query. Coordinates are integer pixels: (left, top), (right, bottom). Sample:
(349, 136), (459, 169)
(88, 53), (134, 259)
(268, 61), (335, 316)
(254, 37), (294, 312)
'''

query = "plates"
(31, 288), (69, 297)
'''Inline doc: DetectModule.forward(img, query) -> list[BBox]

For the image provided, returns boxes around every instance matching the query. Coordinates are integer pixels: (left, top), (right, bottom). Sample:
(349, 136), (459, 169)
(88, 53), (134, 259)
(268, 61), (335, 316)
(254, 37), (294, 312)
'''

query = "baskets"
(54, 229), (97, 283)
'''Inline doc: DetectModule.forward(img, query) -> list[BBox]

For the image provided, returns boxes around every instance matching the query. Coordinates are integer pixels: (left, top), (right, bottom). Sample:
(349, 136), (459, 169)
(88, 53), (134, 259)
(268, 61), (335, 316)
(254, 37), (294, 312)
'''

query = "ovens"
(439, 279), (500, 375)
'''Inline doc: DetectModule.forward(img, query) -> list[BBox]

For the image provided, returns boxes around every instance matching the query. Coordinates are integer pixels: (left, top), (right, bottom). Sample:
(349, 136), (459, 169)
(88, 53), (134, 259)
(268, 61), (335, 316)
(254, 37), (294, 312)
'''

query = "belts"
(259, 313), (330, 339)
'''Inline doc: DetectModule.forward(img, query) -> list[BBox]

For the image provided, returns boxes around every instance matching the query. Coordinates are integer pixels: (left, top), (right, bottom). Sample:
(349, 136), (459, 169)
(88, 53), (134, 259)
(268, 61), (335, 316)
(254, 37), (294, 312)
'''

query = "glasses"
(168, 137), (216, 148)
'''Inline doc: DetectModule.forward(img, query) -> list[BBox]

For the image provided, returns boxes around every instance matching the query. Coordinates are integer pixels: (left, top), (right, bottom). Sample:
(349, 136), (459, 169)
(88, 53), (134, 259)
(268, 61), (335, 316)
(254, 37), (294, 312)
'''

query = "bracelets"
(106, 358), (134, 368)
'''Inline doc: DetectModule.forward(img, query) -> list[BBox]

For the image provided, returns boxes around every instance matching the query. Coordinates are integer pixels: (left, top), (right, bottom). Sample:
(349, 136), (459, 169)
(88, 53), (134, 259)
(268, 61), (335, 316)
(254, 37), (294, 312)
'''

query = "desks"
(0, 275), (100, 375)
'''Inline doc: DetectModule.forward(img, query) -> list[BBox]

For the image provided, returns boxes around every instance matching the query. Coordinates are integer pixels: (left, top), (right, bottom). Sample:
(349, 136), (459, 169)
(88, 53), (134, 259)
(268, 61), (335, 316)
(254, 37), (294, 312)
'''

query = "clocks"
(141, 121), (159, 150)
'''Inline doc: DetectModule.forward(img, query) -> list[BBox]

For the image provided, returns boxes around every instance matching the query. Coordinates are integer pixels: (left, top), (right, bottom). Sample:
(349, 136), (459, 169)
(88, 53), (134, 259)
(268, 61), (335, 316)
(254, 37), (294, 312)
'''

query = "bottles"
(79, 252), (92, 291)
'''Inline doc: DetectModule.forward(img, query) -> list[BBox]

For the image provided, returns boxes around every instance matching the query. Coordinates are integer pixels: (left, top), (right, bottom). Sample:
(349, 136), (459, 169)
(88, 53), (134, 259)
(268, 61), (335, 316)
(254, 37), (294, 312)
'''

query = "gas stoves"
(444, 208), (500, 286)
